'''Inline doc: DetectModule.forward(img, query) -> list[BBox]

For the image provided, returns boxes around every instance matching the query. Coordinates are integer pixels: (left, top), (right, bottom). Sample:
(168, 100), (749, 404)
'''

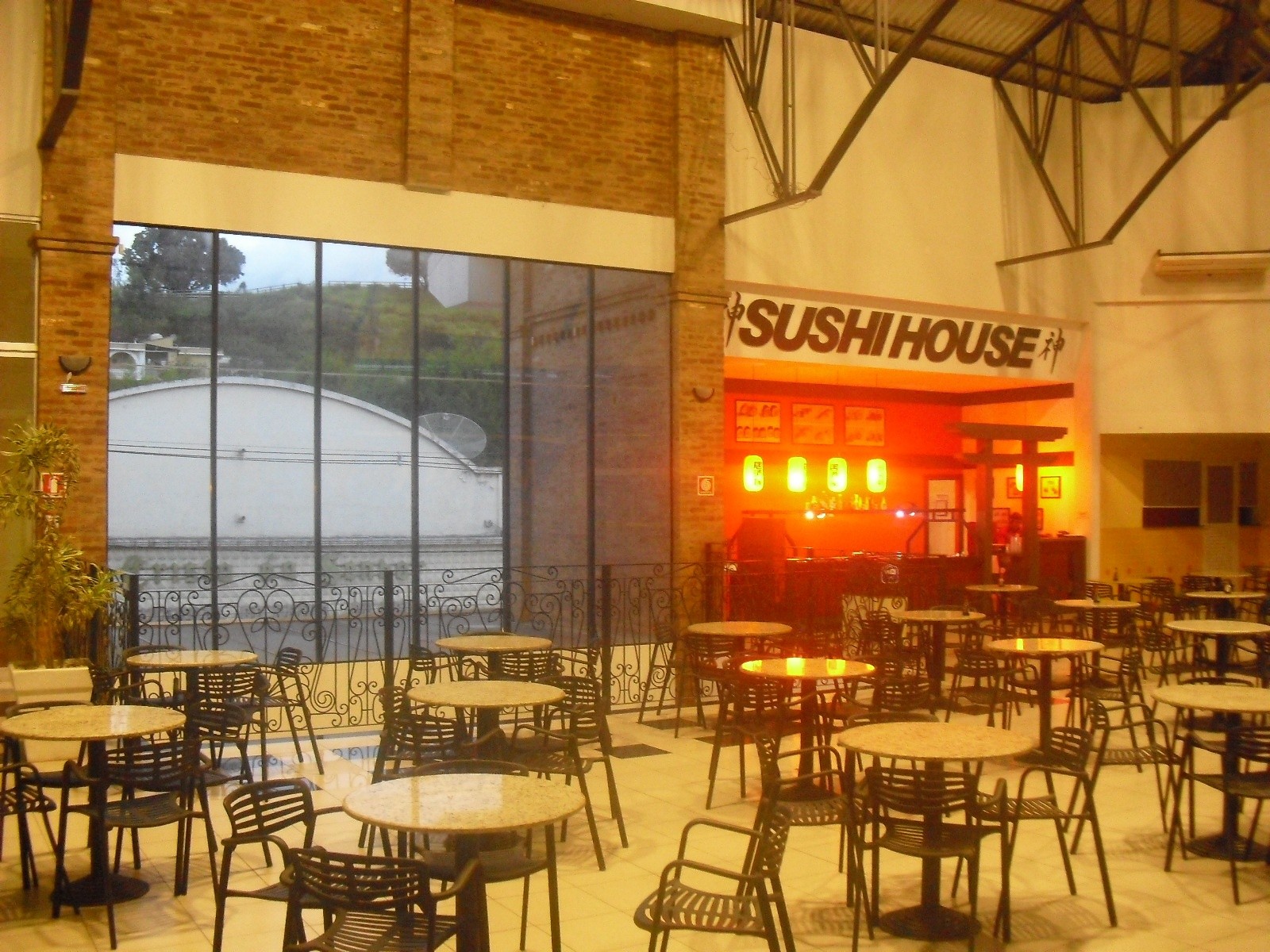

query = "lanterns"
(787, 456), (808, 492)
(743, 453), (764, 492)
(827, 458), (848, 492)
(1015, 464), (1024, 491)
(866, 458), (887, 492)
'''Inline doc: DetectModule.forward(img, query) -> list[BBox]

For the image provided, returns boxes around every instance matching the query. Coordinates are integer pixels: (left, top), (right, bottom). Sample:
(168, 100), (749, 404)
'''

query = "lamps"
(744, 455), (887, 493)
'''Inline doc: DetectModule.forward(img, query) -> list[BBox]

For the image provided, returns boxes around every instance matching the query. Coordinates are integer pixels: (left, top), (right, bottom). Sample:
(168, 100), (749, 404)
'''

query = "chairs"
(0, 576), (1270, 952)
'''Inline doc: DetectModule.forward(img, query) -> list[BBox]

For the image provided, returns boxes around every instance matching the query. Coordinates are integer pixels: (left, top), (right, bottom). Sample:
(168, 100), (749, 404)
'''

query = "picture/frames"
(1007, 476), (1061, 498)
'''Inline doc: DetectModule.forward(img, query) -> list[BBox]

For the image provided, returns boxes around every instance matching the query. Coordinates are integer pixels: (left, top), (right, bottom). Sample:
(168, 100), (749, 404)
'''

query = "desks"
(1167, 620), (1270, 731)
(966, 584), (1038, 638)
(0, 572), (1270, 952)
(2, 706), (186, 909)
(988, 638), (1105, 766)
(739, 658), (876, 812)
(1151, 684), (1270, 861)
(130, 649), (258, 791)
(1185, 591), (1268, 671)
(345, 774), (585, 952)
(838, 722), (1033, 943)
(1055, 600), (1141, 688)
(406, 680), (566, 884)
(897, 610), (986, 708)
(688, 621), (793, 730)
(436, 636), (552, 759)
